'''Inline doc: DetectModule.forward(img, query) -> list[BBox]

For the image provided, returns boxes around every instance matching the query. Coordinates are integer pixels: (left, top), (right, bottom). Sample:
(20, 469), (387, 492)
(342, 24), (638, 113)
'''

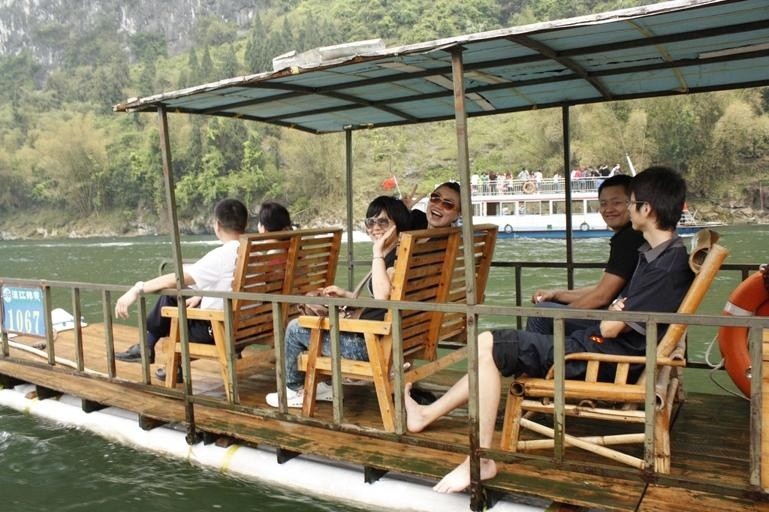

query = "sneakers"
(316, 381), (333, 402)
(265, 387), (304, 408)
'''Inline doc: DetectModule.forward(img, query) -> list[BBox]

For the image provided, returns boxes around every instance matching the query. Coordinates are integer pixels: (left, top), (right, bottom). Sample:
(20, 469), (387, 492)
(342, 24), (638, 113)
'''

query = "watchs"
(135, 281), (145, 295)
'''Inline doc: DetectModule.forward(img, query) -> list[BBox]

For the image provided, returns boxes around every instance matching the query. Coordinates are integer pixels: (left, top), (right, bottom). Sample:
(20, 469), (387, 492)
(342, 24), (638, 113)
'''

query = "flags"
(382, 176), (397, 191)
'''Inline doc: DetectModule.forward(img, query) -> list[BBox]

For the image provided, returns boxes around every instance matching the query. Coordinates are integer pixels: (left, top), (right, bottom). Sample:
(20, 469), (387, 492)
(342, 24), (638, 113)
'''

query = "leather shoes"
(114, 344), (155, 365)
(154, 366), (184, 384)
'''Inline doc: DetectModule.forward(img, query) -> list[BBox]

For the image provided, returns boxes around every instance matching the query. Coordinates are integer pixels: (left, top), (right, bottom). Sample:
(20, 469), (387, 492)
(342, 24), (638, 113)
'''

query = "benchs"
(298, 224), (498, 434)
(159, 228), (343, 405)
(501, 230), (727, 474)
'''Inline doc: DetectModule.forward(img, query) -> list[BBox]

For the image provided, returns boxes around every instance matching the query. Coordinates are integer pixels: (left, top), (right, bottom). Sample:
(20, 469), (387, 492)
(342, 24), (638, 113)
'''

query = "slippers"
(341, 378), (367, 386)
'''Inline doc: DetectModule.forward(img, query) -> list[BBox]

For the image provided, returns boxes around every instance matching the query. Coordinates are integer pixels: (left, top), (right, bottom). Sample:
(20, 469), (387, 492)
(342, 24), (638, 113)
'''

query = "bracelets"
(611, 299), (620, 307)
(372, 256), (385, 261)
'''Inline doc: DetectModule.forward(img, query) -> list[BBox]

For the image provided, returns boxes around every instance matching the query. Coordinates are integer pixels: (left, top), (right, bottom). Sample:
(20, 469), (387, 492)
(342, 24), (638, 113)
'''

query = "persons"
(115, 198), (248, 384)
(409, 181), (461, 245)
(403, 165), (696, 494)
(258, 202), (293, 255)
(265, 196), (412, 409)
(526, 174), (646, 339)
(470, 163), (621, 195)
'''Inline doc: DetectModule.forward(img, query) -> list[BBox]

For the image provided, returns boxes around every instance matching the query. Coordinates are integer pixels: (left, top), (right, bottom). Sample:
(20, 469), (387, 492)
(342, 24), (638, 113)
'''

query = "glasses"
(429, 192), (456, 211)
(365, 217), (391, 229)
(625, 199), (648, 205)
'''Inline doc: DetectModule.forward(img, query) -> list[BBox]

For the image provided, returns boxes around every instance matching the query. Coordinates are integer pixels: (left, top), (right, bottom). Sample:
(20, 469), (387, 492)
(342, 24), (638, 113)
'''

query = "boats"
(406, 174), (725, 240)
(2, 1), (768, 512)
